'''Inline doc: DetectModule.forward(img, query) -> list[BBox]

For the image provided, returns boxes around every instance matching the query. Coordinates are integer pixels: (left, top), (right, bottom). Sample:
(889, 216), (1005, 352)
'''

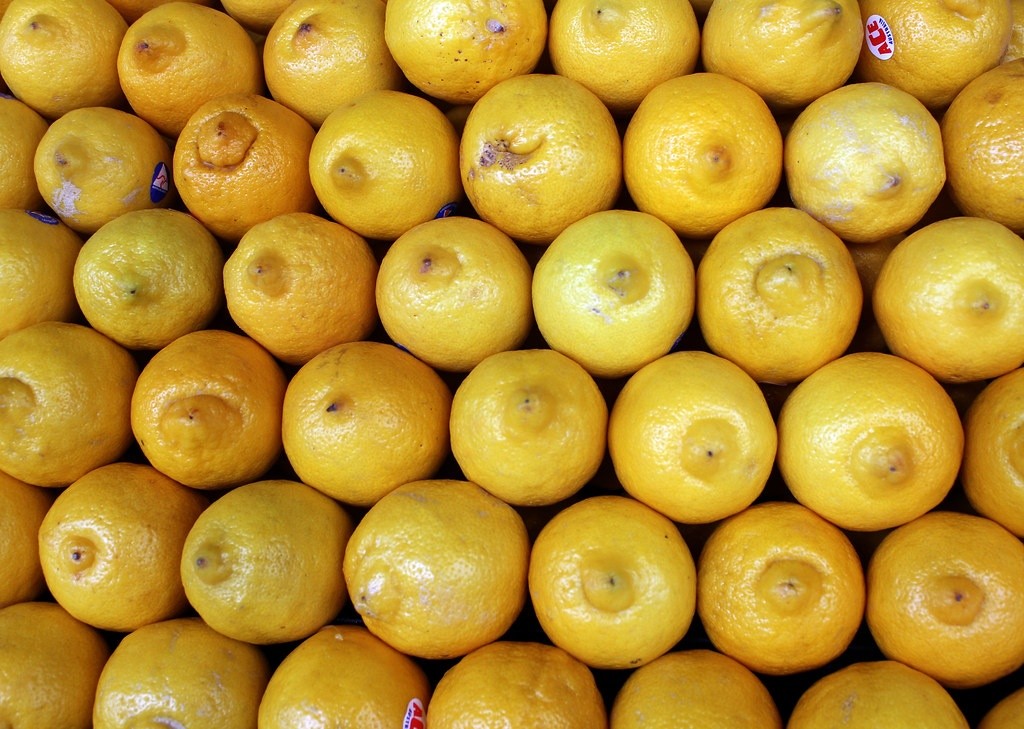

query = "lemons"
(0, 0), (1024, 728)
